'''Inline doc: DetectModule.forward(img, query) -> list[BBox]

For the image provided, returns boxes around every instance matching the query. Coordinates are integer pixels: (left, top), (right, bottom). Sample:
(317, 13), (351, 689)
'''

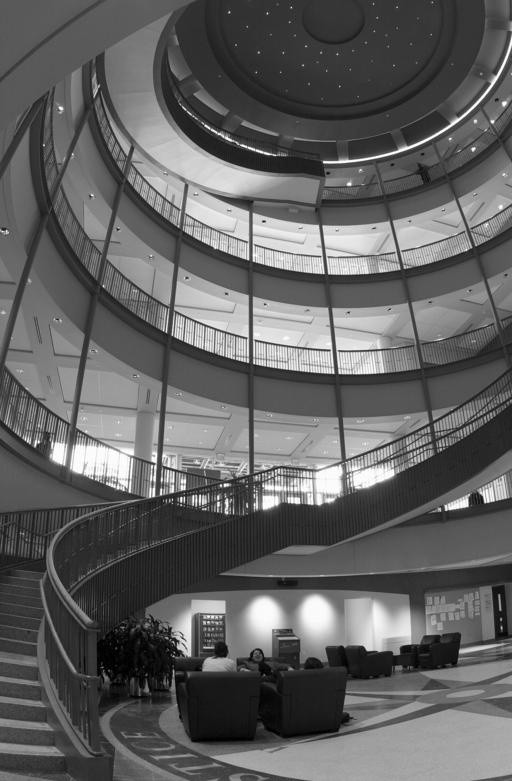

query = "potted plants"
(96, 614), (188, 697)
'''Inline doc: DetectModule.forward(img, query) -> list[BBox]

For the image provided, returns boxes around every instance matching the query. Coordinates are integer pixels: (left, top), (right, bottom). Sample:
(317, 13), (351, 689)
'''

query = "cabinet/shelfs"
(195, 613), (226, 657)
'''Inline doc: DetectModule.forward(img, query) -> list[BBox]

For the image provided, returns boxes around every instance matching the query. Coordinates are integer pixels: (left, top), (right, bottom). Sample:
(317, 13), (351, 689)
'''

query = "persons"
(304, 657), (322, 672)
(248, 649), (271, 676)
(202, 642), (237, 673)
(35, 431), (52, 460)
(416, 162), (432, 184)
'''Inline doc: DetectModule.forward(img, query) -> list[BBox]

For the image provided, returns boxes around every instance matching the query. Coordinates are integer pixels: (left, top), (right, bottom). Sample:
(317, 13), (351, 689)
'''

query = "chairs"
(326, 632), (461, 679)
(172, 656), (349, 742)
